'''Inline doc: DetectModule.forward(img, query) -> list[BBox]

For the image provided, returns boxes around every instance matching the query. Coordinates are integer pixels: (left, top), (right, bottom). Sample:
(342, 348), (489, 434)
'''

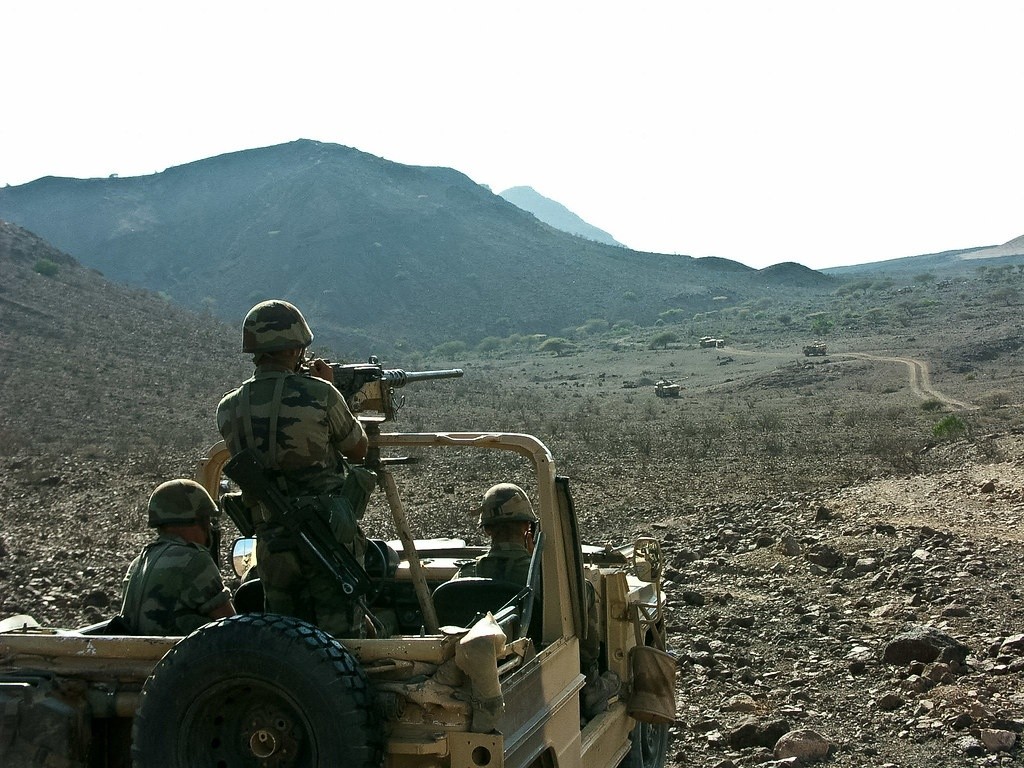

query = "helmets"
(243, 299), (314, 352)
(147, 479), (221, 527)
(478, 483), (539, 529)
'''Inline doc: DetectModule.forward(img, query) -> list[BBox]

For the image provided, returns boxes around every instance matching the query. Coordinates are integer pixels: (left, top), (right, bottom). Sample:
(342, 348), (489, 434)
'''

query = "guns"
(222, 448), (390, 634)
(301, 354), (465, 422)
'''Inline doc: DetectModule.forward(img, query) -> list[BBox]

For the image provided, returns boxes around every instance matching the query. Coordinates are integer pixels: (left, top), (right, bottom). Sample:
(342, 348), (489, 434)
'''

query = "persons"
(452, 484), (621, 717)
(217, 300), (397, 639)
(122, 480), (233, 636)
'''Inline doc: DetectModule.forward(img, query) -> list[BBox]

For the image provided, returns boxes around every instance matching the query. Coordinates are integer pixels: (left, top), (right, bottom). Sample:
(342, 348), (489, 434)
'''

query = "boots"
(583, 659), (621, 720)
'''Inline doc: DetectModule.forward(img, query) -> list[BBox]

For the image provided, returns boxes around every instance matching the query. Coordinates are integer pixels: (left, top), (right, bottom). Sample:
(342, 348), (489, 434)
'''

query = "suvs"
(0, 416), (678, 768)
(802, 343), (827, 357)
(654, 380), (680, 398)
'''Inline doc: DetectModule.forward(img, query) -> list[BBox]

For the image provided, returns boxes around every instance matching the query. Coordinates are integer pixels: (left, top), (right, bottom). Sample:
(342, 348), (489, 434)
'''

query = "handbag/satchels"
(628, 600), (679, 724)
